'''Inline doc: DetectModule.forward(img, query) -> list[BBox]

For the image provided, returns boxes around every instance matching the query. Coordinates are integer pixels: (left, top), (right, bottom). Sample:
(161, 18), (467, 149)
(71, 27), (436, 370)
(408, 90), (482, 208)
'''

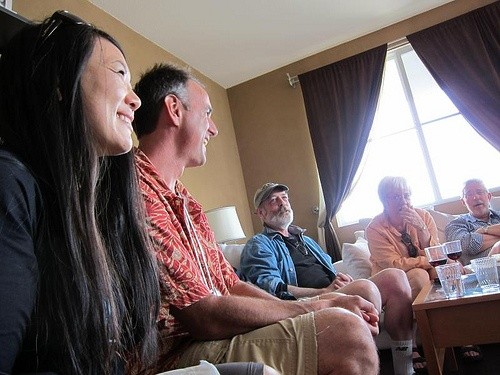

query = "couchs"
(333, 231), (421, 349)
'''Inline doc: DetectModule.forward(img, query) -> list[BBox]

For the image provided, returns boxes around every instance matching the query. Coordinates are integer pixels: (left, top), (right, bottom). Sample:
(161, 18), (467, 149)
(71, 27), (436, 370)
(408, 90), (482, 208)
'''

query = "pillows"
(428, 210), (456, 245)
(342, 237), (371, 280)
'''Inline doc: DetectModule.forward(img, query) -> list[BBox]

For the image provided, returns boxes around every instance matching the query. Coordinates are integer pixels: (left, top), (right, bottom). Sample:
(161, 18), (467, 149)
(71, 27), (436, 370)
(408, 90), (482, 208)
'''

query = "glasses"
(287, 237), (308, 255)
(401, 233), (417, 257)
(38, 10), (88, 40)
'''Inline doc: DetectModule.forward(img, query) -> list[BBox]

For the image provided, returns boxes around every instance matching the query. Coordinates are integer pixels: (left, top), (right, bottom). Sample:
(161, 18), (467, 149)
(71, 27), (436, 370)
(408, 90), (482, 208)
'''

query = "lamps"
(205, 206), (247, 244)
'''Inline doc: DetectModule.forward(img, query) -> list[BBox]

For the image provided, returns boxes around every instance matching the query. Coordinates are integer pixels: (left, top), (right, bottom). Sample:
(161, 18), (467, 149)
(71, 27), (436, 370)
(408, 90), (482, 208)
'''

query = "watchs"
(420, 225), (426, 231)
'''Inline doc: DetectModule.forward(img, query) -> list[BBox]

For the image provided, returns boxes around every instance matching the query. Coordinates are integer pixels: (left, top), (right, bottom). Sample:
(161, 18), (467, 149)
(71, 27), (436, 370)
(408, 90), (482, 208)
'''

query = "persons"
(131, 63), (381, 375)
(241, 183), (431, 375)
(444, 179), (500, 266)
(365, 177), (482, 374)
(0, 9), (279, 375)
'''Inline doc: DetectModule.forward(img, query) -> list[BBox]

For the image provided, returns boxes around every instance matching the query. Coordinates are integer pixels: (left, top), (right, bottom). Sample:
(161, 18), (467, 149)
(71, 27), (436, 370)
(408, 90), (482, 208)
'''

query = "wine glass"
(424, 246), (448, 290)
(443, 240), (463, 263)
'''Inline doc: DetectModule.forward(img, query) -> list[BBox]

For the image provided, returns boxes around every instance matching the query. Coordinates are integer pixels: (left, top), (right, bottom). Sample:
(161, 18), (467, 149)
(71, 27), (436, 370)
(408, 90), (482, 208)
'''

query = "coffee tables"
(412, 266), (500, 375)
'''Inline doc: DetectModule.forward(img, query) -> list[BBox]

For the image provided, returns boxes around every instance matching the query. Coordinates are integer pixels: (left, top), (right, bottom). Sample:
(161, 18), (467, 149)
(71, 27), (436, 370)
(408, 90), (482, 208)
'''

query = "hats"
(253, 183), (290, 208)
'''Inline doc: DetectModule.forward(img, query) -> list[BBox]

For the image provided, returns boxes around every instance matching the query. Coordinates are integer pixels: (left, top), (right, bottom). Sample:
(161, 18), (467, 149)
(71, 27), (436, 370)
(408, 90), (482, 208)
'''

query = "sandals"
(410, 348), (427, 374)
(462, 344), (483, 363)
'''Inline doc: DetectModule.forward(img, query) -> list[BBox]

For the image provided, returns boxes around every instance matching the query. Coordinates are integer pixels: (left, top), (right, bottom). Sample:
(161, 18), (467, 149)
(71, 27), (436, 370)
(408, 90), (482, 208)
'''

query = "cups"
(435, 262), (466, 300)
(470, 256), (500, 295)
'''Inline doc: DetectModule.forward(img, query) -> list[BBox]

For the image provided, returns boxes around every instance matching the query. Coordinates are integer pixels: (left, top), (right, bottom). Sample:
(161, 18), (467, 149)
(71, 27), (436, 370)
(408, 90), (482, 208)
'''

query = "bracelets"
(484, 225), (490, 234)
